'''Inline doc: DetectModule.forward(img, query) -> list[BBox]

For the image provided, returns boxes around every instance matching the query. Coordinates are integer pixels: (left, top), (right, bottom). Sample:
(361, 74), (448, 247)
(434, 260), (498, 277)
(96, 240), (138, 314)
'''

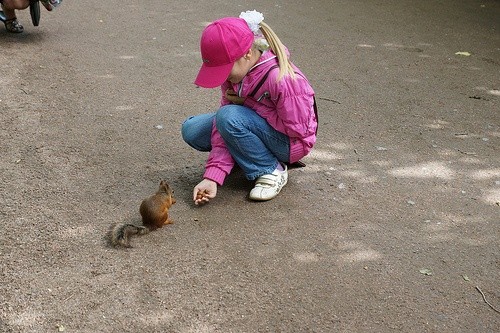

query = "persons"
(0, 0), (62, 34)
(181, 9), (320, 204)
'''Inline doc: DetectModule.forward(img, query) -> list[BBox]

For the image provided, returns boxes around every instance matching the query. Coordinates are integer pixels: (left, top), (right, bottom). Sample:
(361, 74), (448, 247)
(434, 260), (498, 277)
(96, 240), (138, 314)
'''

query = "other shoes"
(248, 161), (289, 200)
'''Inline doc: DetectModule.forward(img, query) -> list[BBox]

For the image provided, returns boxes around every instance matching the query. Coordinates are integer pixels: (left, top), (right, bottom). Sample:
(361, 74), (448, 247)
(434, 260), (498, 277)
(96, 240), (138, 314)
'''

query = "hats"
(193, 16), (255, 88)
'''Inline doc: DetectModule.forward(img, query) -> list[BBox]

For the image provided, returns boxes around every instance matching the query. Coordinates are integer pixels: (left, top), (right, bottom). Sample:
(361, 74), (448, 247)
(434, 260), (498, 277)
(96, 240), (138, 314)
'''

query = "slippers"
(0, 10), (23, 33)
